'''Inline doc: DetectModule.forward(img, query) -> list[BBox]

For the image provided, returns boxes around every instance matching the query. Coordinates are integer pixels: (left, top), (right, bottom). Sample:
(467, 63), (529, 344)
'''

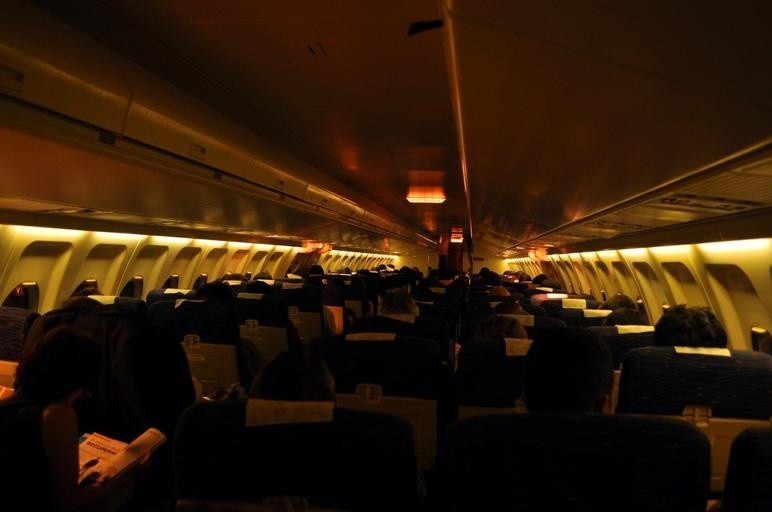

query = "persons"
(1, 265), (728, 511)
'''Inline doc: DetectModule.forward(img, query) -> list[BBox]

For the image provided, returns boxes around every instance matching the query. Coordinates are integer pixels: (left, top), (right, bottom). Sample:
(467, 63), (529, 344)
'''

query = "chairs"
(1, 267), (772, 511)
